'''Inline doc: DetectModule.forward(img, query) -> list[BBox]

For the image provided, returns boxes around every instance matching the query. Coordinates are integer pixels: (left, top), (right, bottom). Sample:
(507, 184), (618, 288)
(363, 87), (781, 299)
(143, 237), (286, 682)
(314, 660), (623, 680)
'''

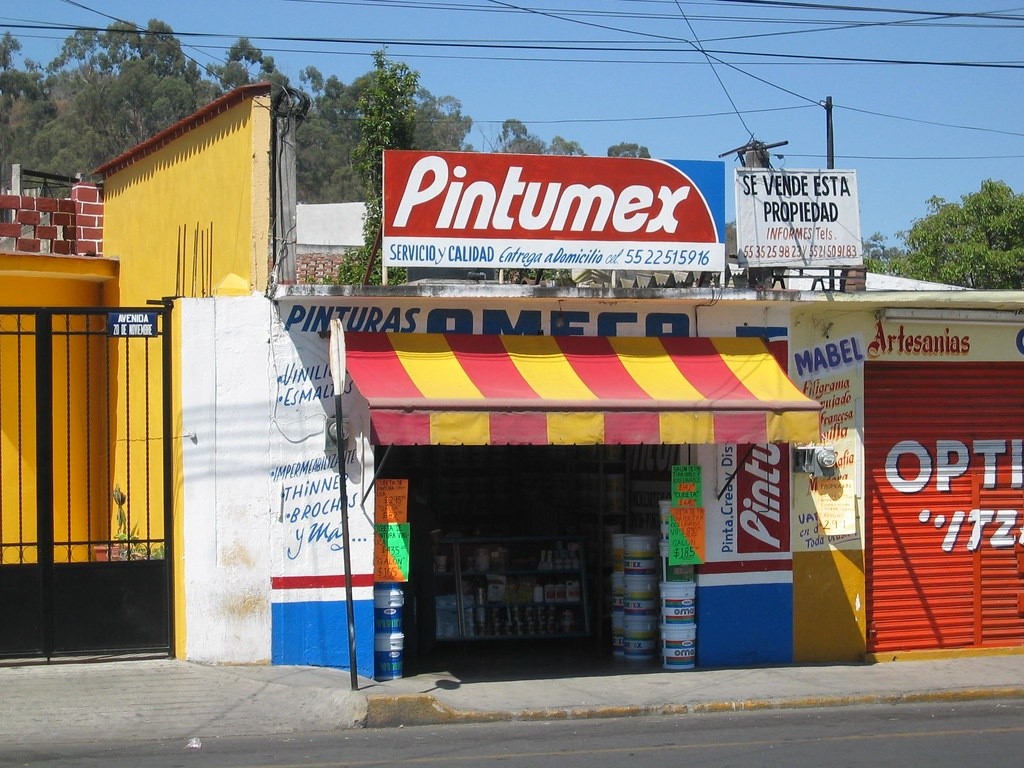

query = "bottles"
(538, 550), (546, 568)
(555, 579), (566, 601)
(567, 580), (580, 601)
(533, 583), (543, 603)
(545, 551), (553, 568)
(544, 579), (555, 602)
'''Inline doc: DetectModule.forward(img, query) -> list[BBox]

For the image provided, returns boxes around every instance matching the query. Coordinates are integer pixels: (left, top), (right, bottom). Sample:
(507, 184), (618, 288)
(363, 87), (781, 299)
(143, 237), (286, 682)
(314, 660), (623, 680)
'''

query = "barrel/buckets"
(660, 624), (696, 671)
(660, 584), (696, 623)
(375, 581), (404, 635)
(626, 574), (662, 615)
(625, 537), (659, 574)
(375, 634), (405, 680)
(660, 543), (696, 583)
(613, 612), (627, 657)
(611, 534), (636, 571)
(612, 573), (626, 613)
(658, 500), (671, 540)
(625, 615), (661, 661)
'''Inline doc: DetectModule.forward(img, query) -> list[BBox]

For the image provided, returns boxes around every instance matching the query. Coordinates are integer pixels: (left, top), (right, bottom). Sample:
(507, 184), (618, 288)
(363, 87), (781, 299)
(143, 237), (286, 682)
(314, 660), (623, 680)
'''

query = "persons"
(401, 490), (440, 657)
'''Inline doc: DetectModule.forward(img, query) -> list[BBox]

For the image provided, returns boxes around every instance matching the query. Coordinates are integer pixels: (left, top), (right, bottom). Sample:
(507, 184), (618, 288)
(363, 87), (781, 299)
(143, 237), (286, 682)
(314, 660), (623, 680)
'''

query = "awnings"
(336, 335), (824, 445)
